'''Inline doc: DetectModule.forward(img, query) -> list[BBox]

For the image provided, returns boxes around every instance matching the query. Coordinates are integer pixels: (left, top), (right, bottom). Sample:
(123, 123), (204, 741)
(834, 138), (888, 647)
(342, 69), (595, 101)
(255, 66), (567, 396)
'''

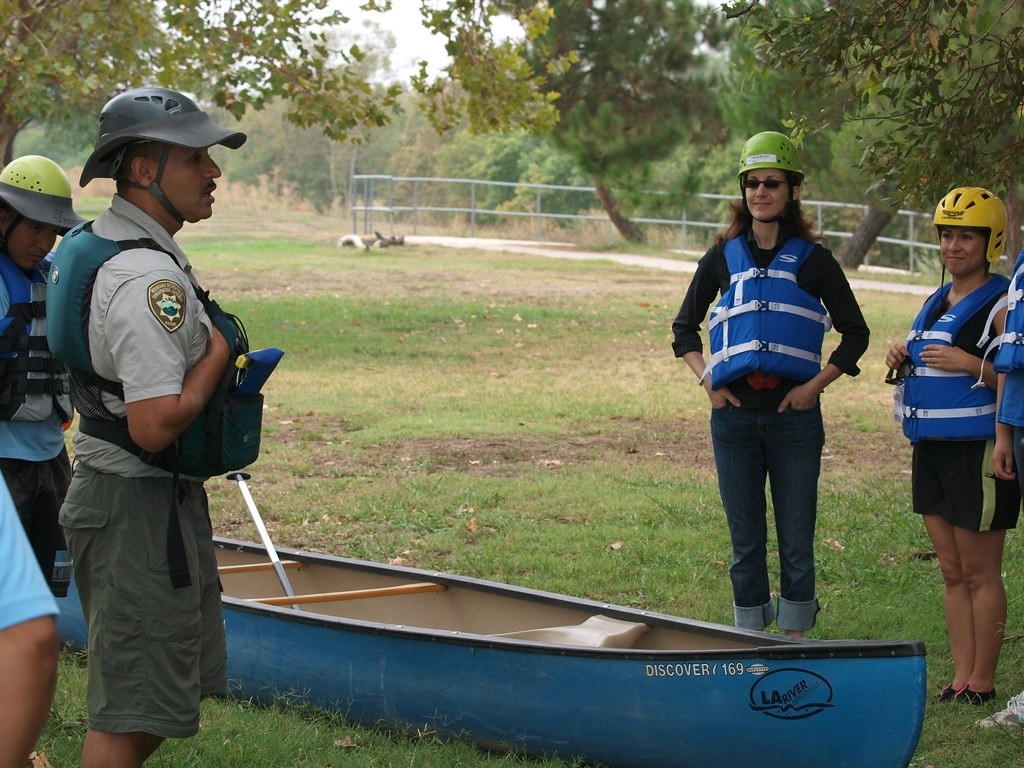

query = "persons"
(671, 133), (870, 640)
(885, 185), (1014, 704)
(0, 465), (60, 768)
(0, 155), (87, 589)
(62, 89), (228, 768)
(976, 248), (1024, 729)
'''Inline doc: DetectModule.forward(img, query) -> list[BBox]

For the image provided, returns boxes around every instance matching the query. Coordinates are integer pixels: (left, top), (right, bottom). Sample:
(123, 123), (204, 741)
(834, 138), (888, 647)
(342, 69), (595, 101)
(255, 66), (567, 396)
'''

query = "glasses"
(741, 179), (788, 189)
(885, 355), (917, 386)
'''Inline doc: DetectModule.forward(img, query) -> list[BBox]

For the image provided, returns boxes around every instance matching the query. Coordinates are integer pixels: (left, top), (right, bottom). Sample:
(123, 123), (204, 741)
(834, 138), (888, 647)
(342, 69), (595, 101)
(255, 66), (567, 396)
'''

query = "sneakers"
(951, 683), (997, 708)
(933, 683), (957, 704)
(974, 690), (1024, 729)
(1003, 709), (1024, 728)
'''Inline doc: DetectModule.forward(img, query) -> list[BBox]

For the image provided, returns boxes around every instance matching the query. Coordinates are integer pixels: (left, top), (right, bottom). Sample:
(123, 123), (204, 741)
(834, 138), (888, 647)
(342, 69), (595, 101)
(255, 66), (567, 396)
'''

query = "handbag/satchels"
(177, 300), (265, 476)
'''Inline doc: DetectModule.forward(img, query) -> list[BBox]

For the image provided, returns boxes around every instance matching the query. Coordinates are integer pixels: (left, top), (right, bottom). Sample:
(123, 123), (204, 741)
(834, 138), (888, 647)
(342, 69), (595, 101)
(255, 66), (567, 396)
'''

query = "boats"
(49, 526), (926, 768)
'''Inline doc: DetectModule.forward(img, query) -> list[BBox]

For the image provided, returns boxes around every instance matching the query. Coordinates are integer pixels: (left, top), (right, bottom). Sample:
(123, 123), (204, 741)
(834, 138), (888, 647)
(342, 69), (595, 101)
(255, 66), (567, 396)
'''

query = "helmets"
(736, 131), (805, 182)
(0, 155), (89, 237)
(933, 186), (1007, 263)
(78, 87), (247, 188)
